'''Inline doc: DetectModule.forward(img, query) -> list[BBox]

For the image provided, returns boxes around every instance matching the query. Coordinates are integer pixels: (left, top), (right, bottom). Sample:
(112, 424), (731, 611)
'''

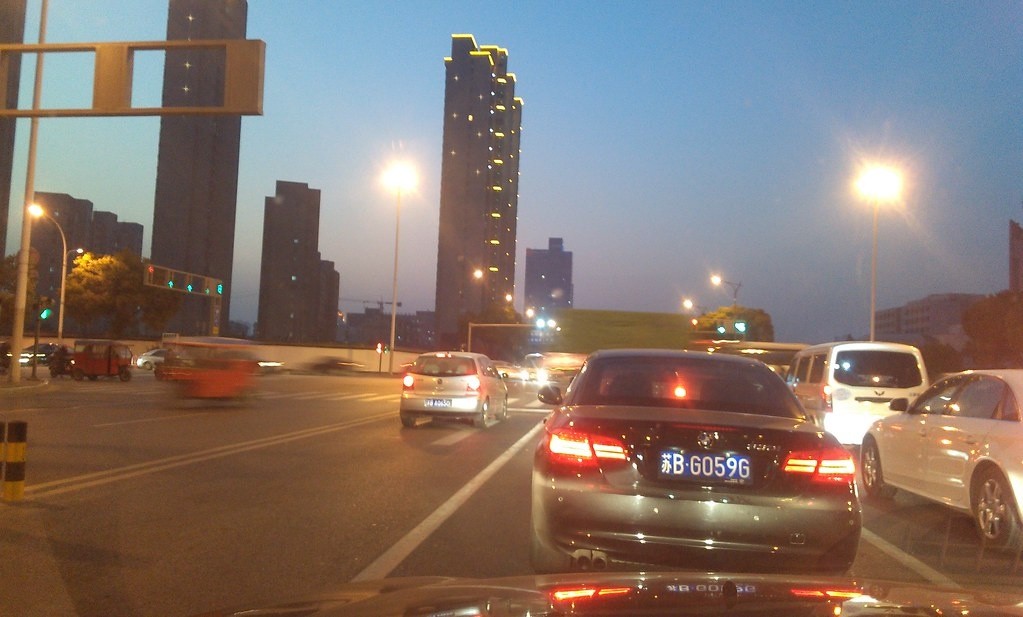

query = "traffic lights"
(147, 264), (153, 285)
(187, 275), (193, 293)
(39, 297), (54, 320)
(216, 279), (223, 295)
(205, 278), (210, 295)
(376, 341), (383, 353)
(168, 270), (174, 288)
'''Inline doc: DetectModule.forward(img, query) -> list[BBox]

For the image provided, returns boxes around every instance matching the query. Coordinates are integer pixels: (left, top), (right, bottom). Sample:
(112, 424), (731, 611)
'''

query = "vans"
(784, 340), (931, 446)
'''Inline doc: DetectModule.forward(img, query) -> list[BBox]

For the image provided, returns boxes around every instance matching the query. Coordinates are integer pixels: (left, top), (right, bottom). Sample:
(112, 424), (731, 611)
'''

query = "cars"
(398, 352), (511, 430)
(21, 342), (60, 366)
(860, 368), (1023, 558)
(491, 359), (529, 380)
(525, 342), (862, 576)
(137, 349), (166, 371)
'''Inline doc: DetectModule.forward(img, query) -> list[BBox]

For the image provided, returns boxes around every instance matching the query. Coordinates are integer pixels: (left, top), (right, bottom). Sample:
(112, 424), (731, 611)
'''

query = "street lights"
(380, 160), (418, 377)
(851, 162), (904, 343)
(710, 276), (742, 345)
(29, 204), (67, 342)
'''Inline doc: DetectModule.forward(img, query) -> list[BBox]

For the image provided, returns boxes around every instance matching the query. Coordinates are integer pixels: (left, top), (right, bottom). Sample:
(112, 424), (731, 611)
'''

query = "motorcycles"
(154, 340), (260, 404)
(71, 341), (133, 382)
(46, 343), (74, 378)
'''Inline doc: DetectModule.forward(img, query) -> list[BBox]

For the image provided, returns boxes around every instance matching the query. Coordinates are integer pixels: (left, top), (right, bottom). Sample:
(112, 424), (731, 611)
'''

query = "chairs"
(457, 365), (468, 372)
(423, 364), (440, 372)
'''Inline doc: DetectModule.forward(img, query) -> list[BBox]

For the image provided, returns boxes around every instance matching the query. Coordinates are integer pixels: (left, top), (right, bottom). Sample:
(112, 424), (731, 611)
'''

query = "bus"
(525, 354), (543, 380)
